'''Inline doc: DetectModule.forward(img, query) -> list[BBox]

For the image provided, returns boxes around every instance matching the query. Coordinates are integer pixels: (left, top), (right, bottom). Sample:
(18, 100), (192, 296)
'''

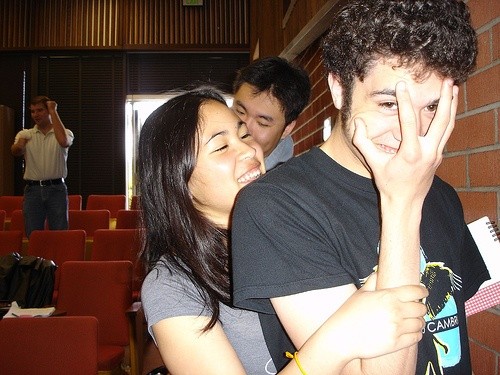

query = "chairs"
(0, 195), (216, 374)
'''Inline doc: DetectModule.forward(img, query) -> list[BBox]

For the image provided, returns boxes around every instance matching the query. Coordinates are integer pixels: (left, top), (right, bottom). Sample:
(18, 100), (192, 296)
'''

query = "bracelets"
(286, 351), (309, 375)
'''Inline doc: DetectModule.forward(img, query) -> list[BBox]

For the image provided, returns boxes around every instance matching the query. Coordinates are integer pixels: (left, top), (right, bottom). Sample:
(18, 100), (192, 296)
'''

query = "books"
(462, 215), (500, 316)
(3, 307), (55, 318)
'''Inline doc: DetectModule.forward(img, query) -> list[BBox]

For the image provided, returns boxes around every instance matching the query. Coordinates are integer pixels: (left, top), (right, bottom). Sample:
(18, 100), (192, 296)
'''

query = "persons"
(11, 96), (73, 239)
(135, 89), (429, 375)
(233, 57), (310, 171)
(230, 0), (492, 375)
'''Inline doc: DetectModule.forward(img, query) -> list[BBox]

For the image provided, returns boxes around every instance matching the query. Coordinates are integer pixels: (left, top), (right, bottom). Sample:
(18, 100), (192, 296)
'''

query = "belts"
(24, 177), (65, 186)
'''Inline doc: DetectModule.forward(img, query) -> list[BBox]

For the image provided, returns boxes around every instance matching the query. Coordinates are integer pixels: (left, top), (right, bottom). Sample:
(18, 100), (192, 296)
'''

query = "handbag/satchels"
(0, 252), (58, 308)
